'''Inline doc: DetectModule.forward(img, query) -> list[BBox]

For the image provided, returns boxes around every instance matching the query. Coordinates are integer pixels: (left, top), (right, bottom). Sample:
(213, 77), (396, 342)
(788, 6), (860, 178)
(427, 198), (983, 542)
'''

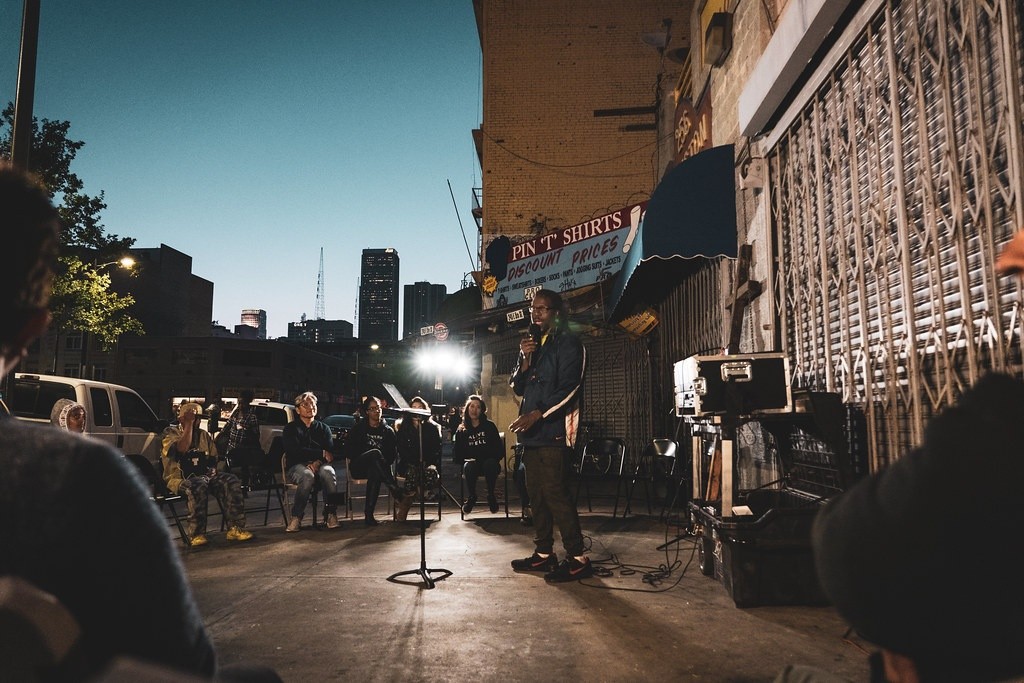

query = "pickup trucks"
(198, 402), (297, 455)
(0, 373), (169, 487)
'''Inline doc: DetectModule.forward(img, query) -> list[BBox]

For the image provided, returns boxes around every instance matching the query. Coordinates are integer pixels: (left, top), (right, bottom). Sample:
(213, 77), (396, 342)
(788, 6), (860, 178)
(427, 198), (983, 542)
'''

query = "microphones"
(528, 324), (541, 366)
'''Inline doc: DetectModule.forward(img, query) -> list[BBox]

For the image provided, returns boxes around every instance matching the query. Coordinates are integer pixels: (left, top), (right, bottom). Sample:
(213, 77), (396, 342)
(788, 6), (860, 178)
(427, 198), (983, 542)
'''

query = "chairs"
(346, 456), (393, 522)
(281, 452), (333, 528)
(393, 443), (442, 522)
(122, 453), (191, 547)
(519, 445), (579, 524)
(623, 437), (681, 520)
(154, 456), (230, 545)
(574, 437), (632, 519)
(220, 451), (288, 533)
(461, 433), (509, 520)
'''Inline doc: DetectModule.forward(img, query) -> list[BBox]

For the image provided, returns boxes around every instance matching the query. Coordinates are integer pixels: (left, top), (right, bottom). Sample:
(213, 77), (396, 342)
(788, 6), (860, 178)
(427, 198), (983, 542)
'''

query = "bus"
(173, 397), (270, 438)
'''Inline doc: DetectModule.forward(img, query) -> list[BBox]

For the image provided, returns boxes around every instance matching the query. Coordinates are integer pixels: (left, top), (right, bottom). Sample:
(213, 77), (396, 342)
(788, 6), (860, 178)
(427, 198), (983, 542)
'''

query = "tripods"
(384, 406), (453, 589)
(656, 435), (711, 550)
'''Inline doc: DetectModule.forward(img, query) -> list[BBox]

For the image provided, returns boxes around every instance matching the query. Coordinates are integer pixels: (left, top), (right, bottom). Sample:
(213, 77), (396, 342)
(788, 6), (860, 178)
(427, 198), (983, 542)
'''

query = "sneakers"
(510, 553), (559, 572)
(543, 556), (593, 583)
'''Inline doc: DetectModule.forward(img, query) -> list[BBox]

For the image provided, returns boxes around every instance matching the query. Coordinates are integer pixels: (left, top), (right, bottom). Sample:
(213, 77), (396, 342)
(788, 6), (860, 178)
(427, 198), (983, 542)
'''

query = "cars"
(322, 414), (399, 444)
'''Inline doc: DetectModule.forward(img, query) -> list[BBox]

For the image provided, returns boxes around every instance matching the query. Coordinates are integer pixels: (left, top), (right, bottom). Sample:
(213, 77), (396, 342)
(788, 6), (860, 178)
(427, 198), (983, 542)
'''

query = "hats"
(178, 403), (209, 421)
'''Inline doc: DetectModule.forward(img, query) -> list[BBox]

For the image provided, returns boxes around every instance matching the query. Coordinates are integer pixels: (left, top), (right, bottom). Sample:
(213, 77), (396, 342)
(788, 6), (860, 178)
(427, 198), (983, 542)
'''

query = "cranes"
(353, 277), (360, 321)
(314, 247), (327, 320)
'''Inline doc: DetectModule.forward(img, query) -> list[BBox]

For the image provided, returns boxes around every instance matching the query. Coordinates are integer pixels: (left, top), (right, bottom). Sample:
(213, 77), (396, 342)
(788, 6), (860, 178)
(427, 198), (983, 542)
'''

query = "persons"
(448, 406), (462, 444)
(49, 397), (88, 436)
(394, 396), (443, 524)
(809, 219), (1024, 683)
(344, 395), (418, 527)
(161, 403), (253, 547)
(454, 394), (506, 515)
(0, 163), (220, 683)
(507, 288), (595, 585)
(282, 391), (340, 532)
(211, 387), (262, 488)
(176, 399), (221, 440)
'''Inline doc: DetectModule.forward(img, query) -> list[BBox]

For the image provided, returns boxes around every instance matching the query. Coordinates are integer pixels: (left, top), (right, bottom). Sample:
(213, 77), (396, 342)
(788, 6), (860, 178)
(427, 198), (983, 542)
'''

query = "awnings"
(603, 142), (739, 327)
(431, 284), (480, 326)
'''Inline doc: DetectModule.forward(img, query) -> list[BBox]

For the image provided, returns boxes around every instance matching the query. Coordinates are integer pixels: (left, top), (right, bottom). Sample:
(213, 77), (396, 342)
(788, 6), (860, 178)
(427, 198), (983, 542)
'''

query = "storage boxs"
(689, 496), (827, 608)
(673, 352), (793, 418)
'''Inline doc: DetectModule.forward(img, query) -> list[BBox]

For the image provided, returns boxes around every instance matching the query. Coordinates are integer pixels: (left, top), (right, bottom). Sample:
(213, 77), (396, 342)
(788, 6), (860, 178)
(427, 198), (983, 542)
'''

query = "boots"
(397, 490), (429, 521)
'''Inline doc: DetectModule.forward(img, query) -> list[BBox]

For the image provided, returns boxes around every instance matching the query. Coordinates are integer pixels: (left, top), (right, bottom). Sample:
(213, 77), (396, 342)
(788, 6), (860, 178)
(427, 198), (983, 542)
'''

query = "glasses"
(367, 406), (381, 410)
(528, 306), (555, 314)
(300, 403), (317, 407)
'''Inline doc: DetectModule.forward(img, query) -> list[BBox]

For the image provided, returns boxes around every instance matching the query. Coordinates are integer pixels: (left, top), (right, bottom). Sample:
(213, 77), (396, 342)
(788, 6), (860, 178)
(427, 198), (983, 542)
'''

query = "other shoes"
(389, 482), (402, 499)
(326, 512), (340, 529)
(227, 526), (253, 540)
(365, 514), (378, 526)
(521, 515), (533, 526)
(462, 495), (477, 515)
(190, 534), (208, 546)
(285, 516), (302, 531)
(486, 495), (499, 514)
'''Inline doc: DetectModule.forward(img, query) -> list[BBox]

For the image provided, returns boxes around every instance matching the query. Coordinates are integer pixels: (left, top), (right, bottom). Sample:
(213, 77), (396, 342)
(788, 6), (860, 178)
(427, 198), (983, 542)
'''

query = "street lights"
(355, 344), (380, 411)
(49, 257), (136, 376)
